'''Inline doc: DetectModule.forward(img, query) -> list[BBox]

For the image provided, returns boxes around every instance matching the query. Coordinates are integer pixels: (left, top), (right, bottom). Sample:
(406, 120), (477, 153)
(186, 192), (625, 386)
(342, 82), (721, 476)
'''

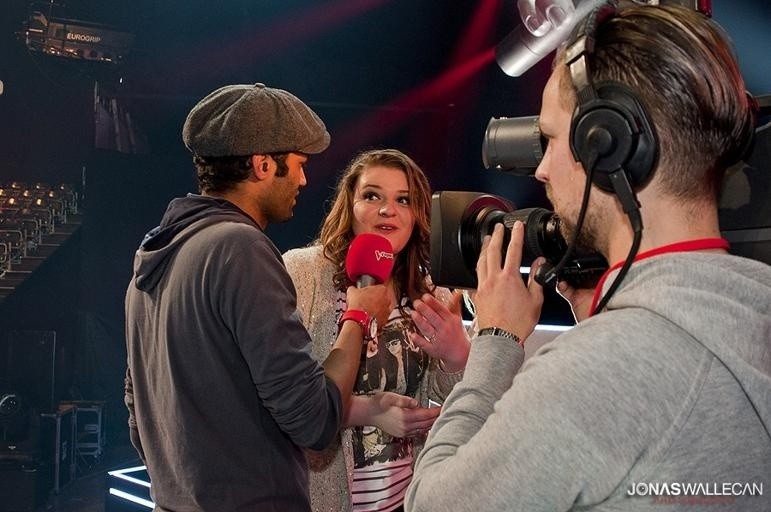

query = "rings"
(427, 332), (436, 342)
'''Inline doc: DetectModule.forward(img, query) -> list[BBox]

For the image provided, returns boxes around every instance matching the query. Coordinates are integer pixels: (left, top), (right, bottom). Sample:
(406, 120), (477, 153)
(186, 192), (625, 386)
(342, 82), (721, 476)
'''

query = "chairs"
(0, 181), (78, 277)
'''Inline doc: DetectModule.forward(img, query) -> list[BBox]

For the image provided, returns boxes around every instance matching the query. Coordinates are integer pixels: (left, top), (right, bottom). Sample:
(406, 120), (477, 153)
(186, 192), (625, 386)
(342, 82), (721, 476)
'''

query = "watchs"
(335, 308), (379, 344)
(475, 327), (521, 343)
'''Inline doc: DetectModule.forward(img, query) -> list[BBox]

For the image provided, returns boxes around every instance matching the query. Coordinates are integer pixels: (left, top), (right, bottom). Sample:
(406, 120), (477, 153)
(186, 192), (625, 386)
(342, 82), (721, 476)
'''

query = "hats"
(182, 82), (332, 157)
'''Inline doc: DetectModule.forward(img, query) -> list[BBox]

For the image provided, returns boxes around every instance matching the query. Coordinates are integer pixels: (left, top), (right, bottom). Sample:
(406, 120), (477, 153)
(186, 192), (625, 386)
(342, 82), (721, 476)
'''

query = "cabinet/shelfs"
(39, 403), (77, 495)
(71, 400), (108, 464)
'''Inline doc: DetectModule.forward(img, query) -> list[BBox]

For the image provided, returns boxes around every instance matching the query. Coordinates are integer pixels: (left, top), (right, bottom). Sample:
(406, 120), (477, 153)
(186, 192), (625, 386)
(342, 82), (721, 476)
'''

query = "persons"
(402, 2), (771, 510)
(277, 147), (476, 512)
(121, 80), (395, 512)
(350, 336), (389, 468)
(383, 330), (419, 460)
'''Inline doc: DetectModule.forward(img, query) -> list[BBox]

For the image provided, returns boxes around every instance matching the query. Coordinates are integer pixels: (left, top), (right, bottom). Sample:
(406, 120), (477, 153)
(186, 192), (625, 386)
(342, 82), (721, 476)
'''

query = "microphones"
(533, 150), (599, 287)
(344, 231), (398, 384)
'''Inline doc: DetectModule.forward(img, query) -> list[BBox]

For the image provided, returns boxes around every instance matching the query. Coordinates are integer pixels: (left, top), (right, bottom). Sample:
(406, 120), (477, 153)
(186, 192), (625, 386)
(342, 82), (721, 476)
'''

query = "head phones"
(560, 19), (661, 196)
